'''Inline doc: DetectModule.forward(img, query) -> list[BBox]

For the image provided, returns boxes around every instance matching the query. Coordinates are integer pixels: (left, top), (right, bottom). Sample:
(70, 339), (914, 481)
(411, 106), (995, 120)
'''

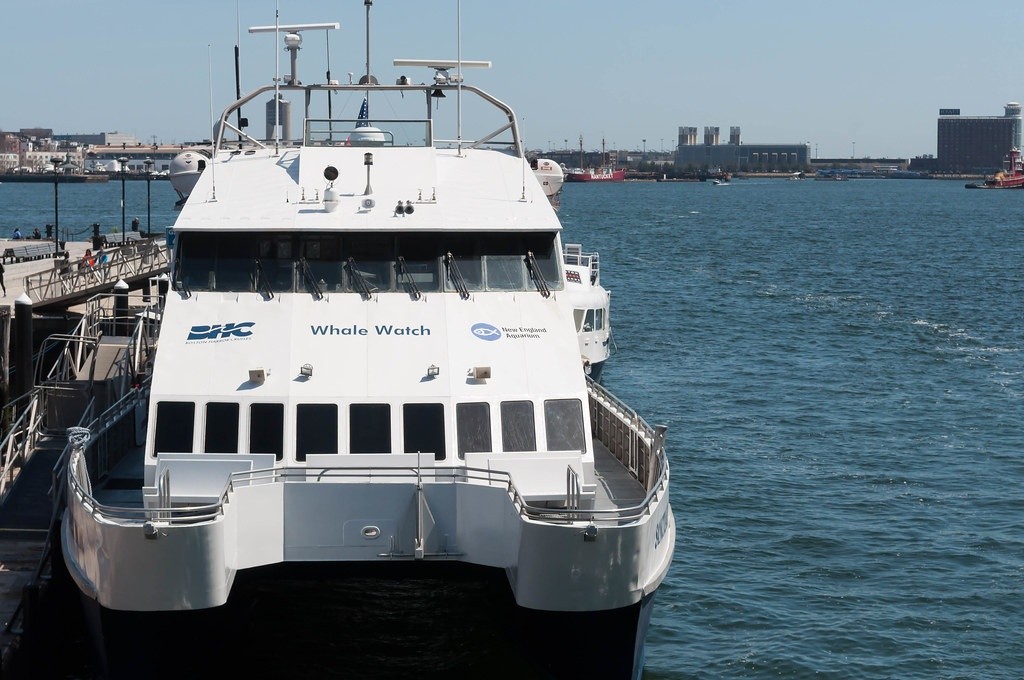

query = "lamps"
(428, 364), (440, 379)
(301, 363), (313, 380)
(364, 152), (374, 195)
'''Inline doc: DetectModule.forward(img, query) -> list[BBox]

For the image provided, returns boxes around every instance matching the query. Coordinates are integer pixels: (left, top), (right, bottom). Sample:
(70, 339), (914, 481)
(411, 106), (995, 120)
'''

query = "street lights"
(116, 155), (130, 244)
(141, 157), (154, 236)
(50, 158), (64, 256)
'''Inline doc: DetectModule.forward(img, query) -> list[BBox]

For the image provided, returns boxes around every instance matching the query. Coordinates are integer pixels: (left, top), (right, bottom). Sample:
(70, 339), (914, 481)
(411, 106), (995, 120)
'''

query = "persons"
(0, 262), (7, 296)
(12, 227), (107, 284)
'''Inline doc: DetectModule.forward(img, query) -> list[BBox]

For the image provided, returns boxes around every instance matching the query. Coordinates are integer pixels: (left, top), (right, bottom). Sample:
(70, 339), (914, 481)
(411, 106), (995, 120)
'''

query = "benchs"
(25, 245), (42, 260)
(37, 243), (55, 259)
(129, 232), (148, 245)
(49, 242), (69, 258)
(13, 247), (32, 263)
(114, 233), (134, 247)
(105, 233), (122, 248)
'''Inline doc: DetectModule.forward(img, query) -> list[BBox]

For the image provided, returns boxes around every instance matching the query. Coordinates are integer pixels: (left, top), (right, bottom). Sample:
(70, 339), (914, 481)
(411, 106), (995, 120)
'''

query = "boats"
(789, 171), (808, 181)
(53, 2), (682, 679)
(814, 169), (849, 182)
(985, 148), (1023, 188)
(562, 135), (627, 181)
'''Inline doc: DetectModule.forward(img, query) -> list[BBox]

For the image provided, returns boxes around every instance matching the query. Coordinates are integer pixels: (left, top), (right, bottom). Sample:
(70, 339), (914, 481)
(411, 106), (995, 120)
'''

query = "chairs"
(53, 260), (74, 294)
(76, 256), (96, 286)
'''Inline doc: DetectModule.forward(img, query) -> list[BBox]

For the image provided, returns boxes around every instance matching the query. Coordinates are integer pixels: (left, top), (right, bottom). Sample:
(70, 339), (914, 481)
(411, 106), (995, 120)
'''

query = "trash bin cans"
(121, 241), (138, 256)
(55, 259), (66, 274)
